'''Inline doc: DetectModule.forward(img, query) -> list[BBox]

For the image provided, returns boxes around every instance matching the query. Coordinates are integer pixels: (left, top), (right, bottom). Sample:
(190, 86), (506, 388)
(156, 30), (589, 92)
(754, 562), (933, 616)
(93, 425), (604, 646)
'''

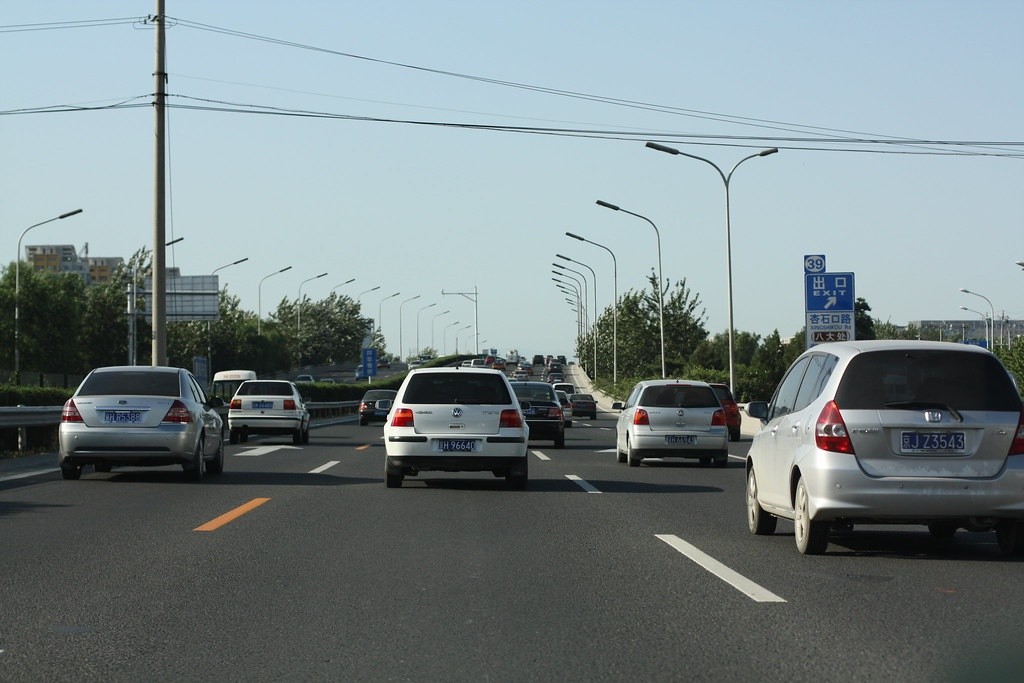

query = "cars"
(611, 376), (729, 467)
(357, 348), (598, 489)
(225, 379), (312, 447)
(706, 382), (743, 442)
(56, 364), (226, 480)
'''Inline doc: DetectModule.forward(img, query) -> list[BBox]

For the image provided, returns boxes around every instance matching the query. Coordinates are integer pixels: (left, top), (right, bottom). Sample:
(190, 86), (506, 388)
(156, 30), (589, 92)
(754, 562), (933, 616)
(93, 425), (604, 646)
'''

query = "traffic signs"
(804, 272), (856, 351)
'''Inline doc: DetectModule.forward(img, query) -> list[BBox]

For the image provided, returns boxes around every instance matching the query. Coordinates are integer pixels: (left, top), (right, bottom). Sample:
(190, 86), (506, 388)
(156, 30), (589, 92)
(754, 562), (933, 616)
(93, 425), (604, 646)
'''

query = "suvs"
(742, 336), (1024, 559)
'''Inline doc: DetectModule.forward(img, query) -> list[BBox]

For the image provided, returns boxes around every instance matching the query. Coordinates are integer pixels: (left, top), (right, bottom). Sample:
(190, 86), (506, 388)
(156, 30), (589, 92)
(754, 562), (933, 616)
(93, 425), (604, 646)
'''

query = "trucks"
(211, 370), (258, 409)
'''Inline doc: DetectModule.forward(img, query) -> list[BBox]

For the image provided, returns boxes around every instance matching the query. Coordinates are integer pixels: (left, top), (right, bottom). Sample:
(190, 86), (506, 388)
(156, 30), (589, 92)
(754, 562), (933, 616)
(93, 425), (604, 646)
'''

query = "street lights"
(960, 287), (995, 356)
(11, 206), (489, 382)
(547, 197), (667, 387)
(645, 139), (778, 405)
